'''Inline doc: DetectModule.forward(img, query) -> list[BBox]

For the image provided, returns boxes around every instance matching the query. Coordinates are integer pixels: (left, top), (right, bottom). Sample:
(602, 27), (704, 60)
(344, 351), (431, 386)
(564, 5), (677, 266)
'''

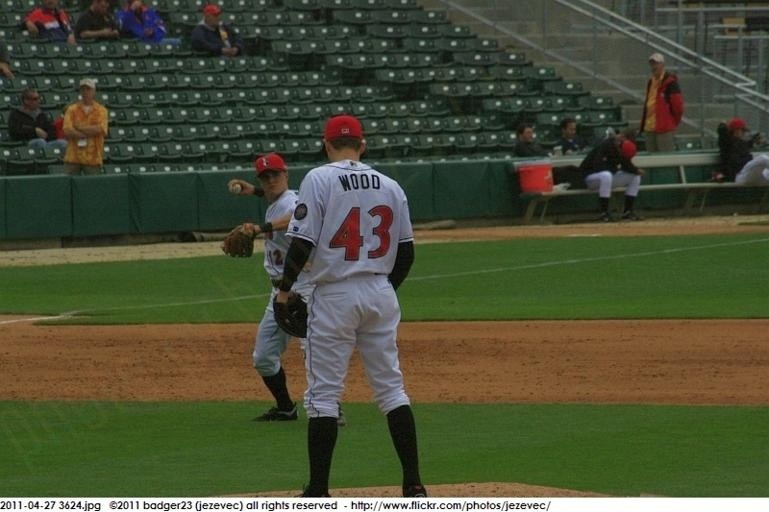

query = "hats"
(649, 53), (664, 63)
(79, 78), (95, 88)
(322, 115), (362, 153)
(621, 140), (637, 158)
(204, 5), (220, 16)
(255, 154), (287, 177)
(729, 119), (750, 131)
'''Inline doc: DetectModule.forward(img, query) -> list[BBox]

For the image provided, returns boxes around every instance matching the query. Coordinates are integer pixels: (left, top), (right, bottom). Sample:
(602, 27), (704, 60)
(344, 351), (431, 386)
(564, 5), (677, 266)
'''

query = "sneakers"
(254, 401), (299, 421)
(622, 212), (639, 220)
(337, 402), (346, 426)
(403, 483), (426, 497)
(295, 483), (331, 497)
(600, 211), (613, 222)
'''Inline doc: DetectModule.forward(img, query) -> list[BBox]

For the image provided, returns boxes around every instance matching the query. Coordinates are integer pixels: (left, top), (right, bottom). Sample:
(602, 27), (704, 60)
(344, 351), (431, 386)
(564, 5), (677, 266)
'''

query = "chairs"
(0, 0), (703, 175)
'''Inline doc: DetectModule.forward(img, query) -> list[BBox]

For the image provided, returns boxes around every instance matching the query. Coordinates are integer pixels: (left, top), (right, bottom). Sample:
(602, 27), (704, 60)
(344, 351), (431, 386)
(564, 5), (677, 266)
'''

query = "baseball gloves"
(221, 222), (256, 258)
(273, 290), (309, 335)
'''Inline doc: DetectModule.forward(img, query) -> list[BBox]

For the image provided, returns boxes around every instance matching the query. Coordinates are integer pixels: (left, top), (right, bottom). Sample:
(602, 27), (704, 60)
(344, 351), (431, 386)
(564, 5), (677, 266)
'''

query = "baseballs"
(228, 183), (241, 195)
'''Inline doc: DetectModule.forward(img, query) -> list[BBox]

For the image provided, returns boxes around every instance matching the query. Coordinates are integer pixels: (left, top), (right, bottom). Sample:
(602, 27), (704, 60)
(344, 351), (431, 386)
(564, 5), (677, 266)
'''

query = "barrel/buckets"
(515, 163), (553, 195)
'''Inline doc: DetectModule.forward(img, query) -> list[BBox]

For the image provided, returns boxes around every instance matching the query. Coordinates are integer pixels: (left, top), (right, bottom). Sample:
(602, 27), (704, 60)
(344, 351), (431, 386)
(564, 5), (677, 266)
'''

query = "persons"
(0, 0), (768, 223)
(221, 154), (347, 427)
(274, 116), (427, 496)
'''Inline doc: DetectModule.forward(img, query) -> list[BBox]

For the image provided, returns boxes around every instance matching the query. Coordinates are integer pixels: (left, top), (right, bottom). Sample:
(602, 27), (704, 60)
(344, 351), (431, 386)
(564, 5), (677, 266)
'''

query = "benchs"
(512, 151), (768, 222)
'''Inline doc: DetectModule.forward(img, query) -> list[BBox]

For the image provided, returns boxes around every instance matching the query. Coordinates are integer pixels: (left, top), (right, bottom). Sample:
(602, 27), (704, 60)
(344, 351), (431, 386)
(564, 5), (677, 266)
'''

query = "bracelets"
(255, 187), (265, 197)
(258, 223), (273, 232)
